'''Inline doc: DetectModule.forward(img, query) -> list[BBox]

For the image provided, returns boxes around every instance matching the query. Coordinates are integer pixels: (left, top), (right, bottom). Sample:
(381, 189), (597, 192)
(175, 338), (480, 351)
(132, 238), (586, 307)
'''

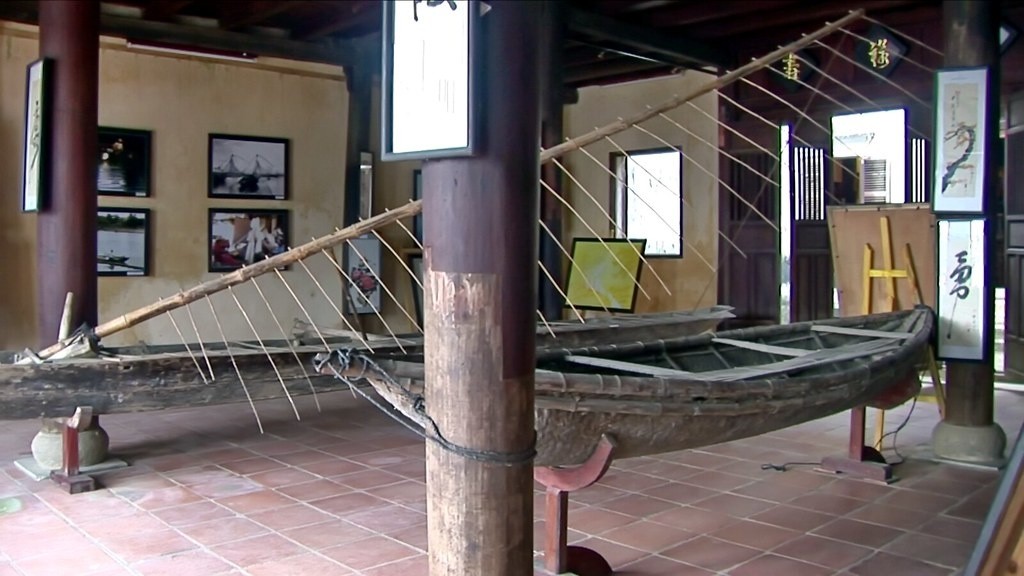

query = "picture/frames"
(930, 65), (988, 215)
(343, 234), (383, 315)
(98, 205), (153, 278)
(208, 208), (290, 274)
(381, 0), (479, 161)
(935, 215), (992, 364)
(91, 127), (153, 198)
(21, 55), (50, 214)
(207, 133), (291, 200)
(561, 237), (647, 313)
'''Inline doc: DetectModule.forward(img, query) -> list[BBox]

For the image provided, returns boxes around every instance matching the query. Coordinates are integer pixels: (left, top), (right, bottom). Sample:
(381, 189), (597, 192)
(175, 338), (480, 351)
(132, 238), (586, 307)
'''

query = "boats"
(238, 175), (259, 189)
(97, 255), (129, 263)
(347, 304), (740, 365)
(310, 290), (936, 468)
